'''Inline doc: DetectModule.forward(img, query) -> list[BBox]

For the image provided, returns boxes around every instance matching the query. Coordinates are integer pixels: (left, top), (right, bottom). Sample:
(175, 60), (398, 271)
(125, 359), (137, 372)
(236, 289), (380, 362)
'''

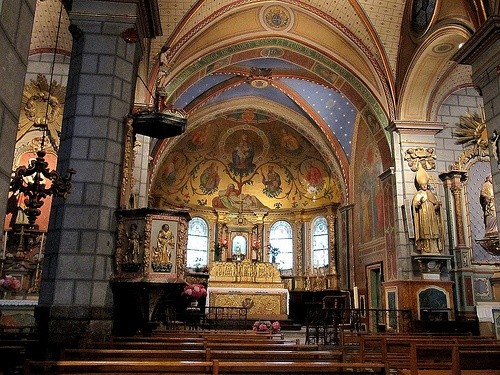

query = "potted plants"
(193, 256), (204, 272)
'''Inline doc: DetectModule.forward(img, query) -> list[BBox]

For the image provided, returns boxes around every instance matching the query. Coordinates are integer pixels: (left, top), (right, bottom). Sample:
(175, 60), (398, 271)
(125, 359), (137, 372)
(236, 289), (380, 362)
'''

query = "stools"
(183, 310), (207, 333)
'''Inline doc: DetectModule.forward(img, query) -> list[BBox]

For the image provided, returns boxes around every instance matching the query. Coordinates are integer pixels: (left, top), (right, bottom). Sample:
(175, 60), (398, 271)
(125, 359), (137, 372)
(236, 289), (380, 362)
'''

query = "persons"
(156, 224), (175, 264)
(414, 168), (442, 254)
(482, 174), (498, 234)
(125, 223), (139, 263)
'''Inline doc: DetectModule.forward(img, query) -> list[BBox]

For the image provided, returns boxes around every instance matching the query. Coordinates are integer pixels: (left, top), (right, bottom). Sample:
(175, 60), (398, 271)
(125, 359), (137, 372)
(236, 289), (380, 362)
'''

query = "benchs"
(0, 329), (500, 375)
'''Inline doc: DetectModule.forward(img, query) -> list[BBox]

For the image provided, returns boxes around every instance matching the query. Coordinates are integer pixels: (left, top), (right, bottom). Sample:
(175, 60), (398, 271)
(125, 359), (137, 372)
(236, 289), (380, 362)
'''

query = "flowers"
(250, 240), (262, 255)
(0, 274), (21, 289)
(252, 320), (281, 334)
(203, 266), (209, 271)
(209, 239), (229, 254)
(266, 245), (281, 257)
(182, 283), (208, 298)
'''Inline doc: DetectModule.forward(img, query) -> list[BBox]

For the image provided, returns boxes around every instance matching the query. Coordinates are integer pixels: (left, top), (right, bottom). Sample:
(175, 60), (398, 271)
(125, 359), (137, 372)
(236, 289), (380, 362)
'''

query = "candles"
(2, 231), (9, 259)
(38, 232), (45, 260)
(353, 286), (359, 309)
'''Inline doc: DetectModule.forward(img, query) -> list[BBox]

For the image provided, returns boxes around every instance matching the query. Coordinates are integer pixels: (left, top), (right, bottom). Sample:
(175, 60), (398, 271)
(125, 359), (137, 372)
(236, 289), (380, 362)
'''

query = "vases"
(191, 298), (199, 307)
(272, 256), (275, 263)
(203, 270), (208, 273)
(255, 332), (274, 340)
(214, 251), (222, 261)
(255, 251), (261, 261)
